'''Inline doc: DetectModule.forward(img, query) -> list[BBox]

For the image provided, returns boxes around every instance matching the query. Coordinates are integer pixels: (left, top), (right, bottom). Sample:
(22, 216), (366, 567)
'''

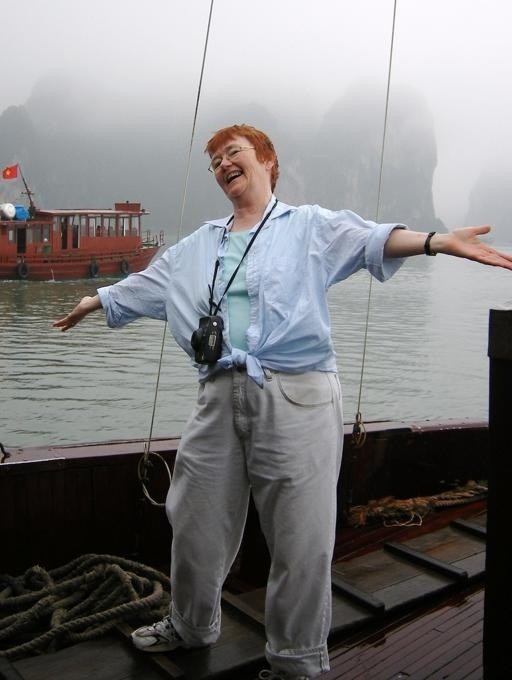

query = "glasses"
(207, 145), (256, 174)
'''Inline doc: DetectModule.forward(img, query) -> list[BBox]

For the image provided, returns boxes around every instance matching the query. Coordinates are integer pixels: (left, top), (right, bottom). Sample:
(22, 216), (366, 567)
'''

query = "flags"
(3, 163), (19, 180)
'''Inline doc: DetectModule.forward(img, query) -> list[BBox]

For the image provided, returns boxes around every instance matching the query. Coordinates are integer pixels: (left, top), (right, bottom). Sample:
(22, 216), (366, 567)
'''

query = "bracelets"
(423, 230), (437, 257)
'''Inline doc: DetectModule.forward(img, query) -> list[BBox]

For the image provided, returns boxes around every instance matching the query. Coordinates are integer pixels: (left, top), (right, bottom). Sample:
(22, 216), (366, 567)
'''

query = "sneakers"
(127, 614), (212, 653)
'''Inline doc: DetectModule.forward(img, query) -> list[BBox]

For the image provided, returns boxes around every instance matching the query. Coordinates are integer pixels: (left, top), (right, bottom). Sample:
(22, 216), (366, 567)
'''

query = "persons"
(95, 224), (125, 236)
(53, 120), (511, 680)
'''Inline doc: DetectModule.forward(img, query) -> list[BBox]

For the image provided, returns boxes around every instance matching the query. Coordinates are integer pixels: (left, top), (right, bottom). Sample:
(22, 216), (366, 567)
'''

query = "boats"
(0, 160), (165, 281)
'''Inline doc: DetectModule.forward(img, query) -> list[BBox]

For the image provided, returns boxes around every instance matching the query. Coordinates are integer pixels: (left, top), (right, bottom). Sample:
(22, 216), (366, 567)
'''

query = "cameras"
(190, 316), (224, 366)
